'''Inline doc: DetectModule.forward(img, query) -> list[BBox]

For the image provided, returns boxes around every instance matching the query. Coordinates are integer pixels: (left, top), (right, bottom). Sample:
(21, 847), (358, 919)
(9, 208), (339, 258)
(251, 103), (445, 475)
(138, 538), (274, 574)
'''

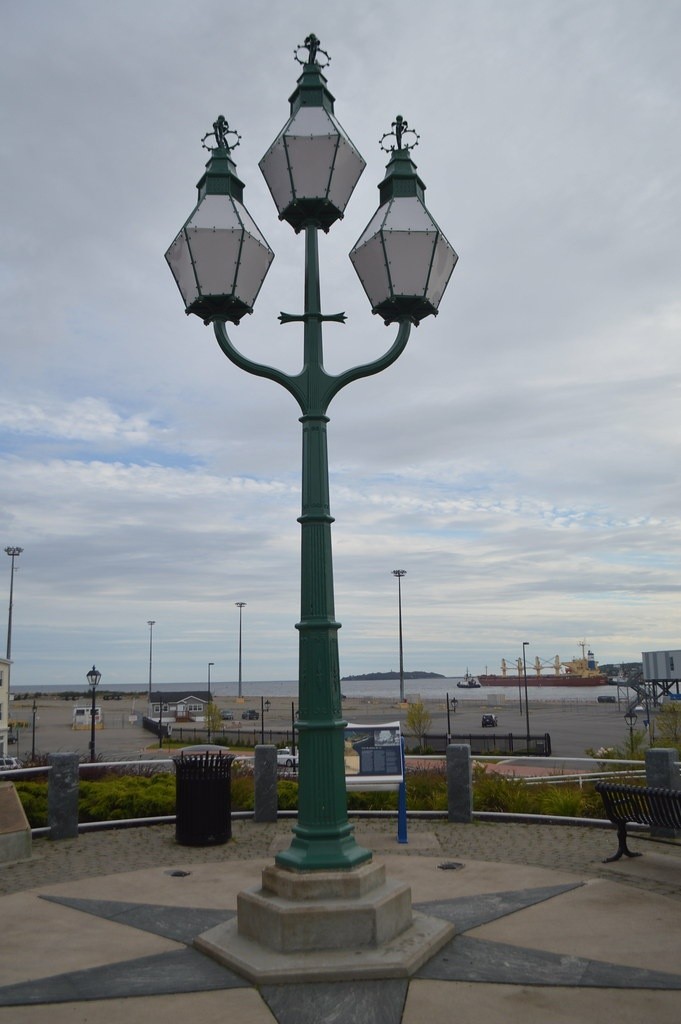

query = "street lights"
(390, 569), (408, 703)
(445, 692), (458, 745)
(157, 32), (460, 1024)
(233, 601), (247, 699)
(291, 700), (299, 773)
(522, 641), (531, 755)
(208, 662), (215, 693)
(147, 620), (155, 699)
(4, 545), (24, 668)
(31, 699), (39, 760)
(260, 695), (271, 745)
(86, 666), (102, 765)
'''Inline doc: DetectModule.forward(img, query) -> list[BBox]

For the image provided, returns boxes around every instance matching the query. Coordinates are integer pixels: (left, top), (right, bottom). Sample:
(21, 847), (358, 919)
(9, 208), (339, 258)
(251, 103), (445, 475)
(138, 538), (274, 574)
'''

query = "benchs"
(595, 780), (681, 863)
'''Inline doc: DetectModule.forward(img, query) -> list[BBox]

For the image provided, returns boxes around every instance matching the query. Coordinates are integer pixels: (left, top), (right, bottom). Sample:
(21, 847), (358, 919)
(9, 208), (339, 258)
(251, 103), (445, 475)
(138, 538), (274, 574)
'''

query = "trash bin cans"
(168, 745), (238, 847)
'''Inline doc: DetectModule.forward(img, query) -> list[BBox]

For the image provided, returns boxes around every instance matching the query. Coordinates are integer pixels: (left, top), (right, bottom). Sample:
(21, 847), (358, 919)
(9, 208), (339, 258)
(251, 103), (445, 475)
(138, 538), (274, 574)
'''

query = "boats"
(457, 679), (481, 688)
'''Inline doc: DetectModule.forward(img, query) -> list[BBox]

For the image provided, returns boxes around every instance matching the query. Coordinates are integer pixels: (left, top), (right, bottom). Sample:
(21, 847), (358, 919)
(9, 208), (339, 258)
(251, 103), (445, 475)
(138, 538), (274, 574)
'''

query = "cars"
(481, 714), (497, 728)
(275, 748), (300, 767)
(241, 709), (259, 720)
(219, 709), (233, 721)
